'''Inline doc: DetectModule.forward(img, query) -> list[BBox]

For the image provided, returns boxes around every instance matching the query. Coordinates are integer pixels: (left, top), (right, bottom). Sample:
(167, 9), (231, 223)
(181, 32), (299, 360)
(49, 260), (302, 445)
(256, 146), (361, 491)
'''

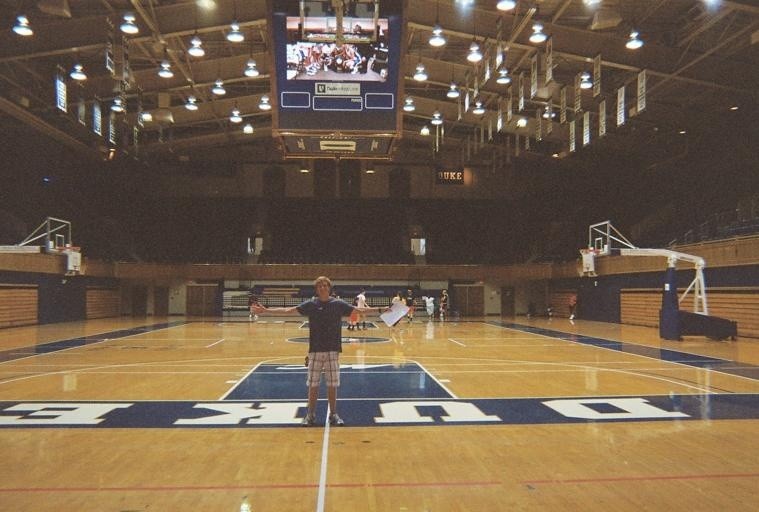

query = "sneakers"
(329, 413), (345, 426)
(348, 327), (350, 330)
(363, 328), (367, 330)
(569, 315), (576, 320)
(357, 328), (361, 331)
(302, 412), (316, 427)
(352, 328), (355, 330)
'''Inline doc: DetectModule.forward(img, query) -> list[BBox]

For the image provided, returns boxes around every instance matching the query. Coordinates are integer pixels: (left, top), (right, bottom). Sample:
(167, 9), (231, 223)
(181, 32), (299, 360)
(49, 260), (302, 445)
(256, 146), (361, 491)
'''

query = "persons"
(568, 319), (577, 345)
(251, 275), (394, 427)
(347, 286), (449, 331)
(249, 291), (259, 319)
(568, 291), (578, 321)
(344, 320), (449, 372)
(294, 24), (370, 78)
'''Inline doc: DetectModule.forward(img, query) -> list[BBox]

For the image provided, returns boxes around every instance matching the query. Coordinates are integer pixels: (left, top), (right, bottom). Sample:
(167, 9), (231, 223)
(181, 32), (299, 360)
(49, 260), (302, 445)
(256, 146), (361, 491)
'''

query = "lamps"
(403, 0), (644, 136)
(11, 3), (273, 135)
